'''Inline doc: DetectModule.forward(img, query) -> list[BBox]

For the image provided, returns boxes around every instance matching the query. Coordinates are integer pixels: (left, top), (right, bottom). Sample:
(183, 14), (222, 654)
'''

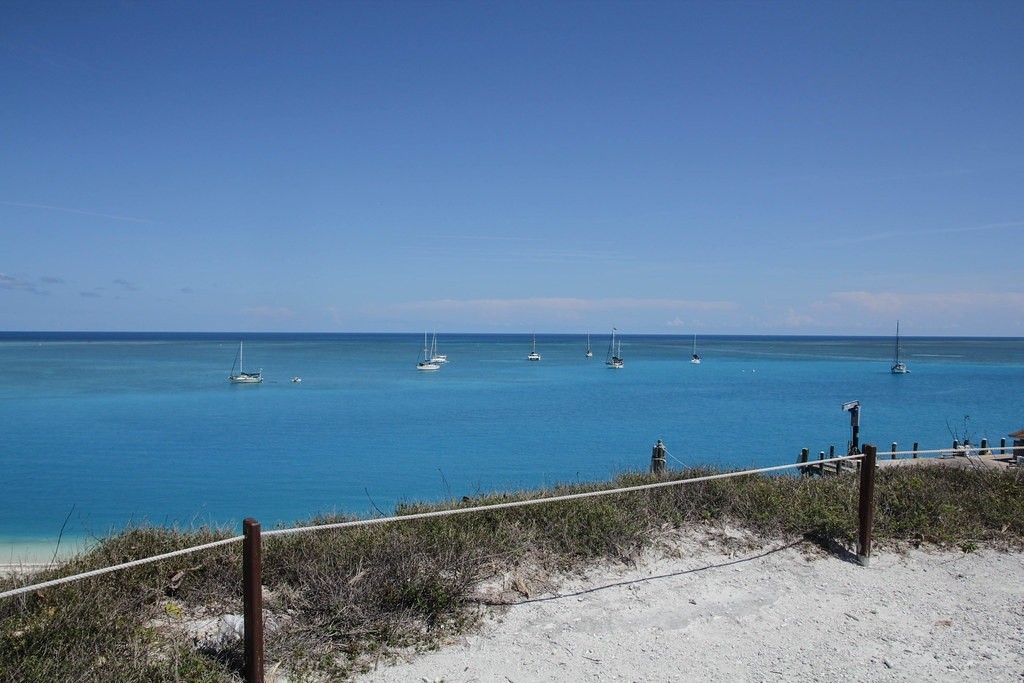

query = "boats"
(292, 376), (301, 382)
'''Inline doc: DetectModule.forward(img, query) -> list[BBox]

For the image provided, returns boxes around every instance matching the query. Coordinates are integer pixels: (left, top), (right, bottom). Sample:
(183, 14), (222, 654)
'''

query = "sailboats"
(691, 332), (700, 364)
(605, 327), (624, 369)
(429, 329), (446, 363)
(528, 330), (540, 360)
(891, 319), (907, 373)
(228, 340), (263, 383)
(416, 329), (440, 370)
(586, 333), (592, 357)
